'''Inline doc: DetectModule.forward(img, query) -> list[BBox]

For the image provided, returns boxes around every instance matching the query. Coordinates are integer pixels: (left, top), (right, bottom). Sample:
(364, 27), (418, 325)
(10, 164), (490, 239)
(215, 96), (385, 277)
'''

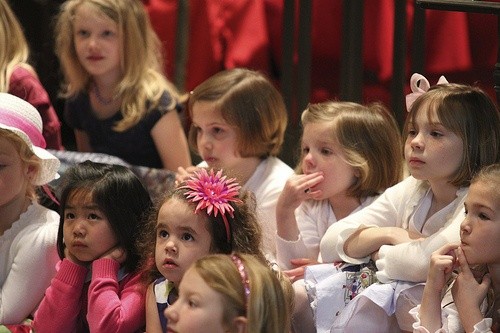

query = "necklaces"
(91, 78), (113, 105)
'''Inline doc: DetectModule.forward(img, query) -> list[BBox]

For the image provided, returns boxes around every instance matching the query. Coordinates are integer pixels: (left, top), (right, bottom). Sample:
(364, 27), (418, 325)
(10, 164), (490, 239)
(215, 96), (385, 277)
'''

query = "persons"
(54, 0), (194, 174)
(0, 67), (500, 333)
(0, 0), (65, 152)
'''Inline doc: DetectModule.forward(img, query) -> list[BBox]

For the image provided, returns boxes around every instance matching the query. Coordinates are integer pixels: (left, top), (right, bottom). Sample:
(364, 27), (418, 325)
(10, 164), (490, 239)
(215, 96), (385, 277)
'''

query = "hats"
(0, 93), (60, 186)
